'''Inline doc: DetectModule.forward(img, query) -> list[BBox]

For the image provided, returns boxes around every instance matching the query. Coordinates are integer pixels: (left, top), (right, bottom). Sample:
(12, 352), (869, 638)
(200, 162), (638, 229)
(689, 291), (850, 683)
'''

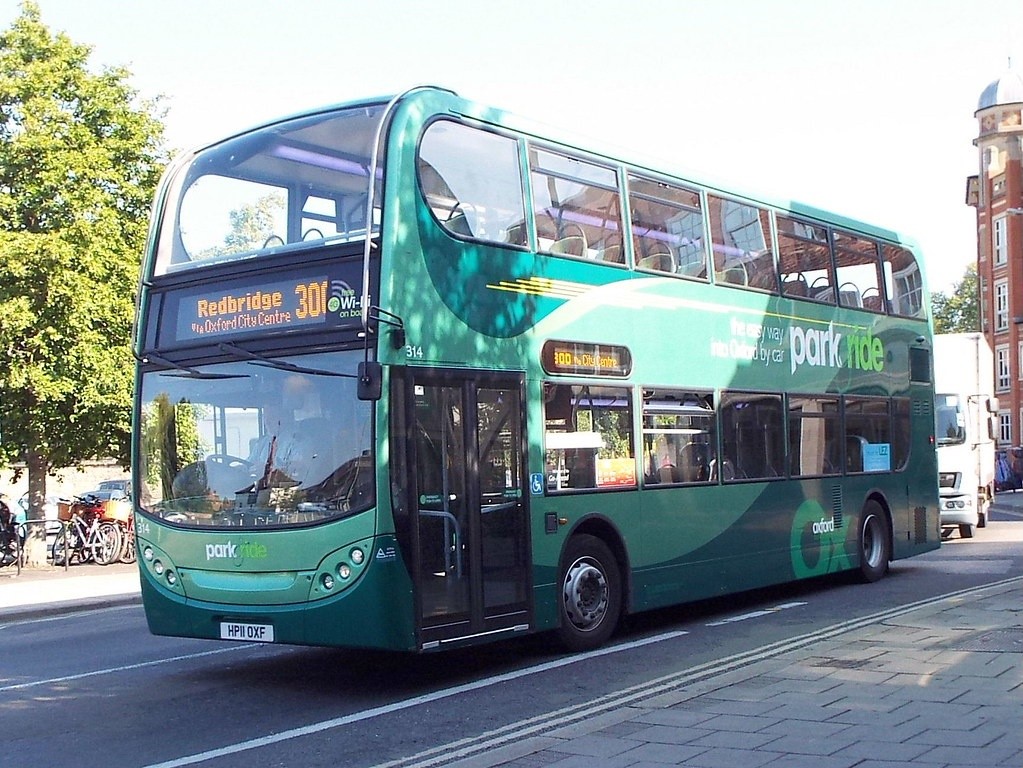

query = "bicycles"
(0, 523), (26, 567)
(53, 493), (138, 567)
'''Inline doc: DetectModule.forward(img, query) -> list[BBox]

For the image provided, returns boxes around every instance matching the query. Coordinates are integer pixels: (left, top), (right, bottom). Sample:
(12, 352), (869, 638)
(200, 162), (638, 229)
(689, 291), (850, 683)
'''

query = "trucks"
(933, 332), (1000, 537)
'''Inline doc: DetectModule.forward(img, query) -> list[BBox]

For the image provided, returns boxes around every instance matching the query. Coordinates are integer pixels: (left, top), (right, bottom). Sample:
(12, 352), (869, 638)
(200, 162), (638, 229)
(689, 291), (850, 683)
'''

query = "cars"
(18, 491), (66, 527)
(75, 479), (147, 501)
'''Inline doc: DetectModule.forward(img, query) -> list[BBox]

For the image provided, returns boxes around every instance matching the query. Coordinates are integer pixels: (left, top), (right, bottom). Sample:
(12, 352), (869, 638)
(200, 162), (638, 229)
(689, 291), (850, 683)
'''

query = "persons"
(235, 402), (310, 480)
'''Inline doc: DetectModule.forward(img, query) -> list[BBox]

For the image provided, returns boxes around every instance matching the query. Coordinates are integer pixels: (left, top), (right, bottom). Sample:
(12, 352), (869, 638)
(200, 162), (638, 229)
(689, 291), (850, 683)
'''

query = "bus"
(130, 85), (941, 656)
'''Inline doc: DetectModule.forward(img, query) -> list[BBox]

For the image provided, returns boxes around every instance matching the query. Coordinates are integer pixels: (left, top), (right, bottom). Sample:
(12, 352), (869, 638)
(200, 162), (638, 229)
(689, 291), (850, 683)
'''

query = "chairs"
(262, 235), (285, 249)
(301, 227), (324, 243)
(643, 457), (834, 487)
(439, 200), (886, 314)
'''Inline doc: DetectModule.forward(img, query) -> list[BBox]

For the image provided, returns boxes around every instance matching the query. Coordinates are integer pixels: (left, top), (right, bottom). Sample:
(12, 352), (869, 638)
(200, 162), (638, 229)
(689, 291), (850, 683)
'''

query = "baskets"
(102, 500), (132, 523)
(58, 502), (86, 522)
